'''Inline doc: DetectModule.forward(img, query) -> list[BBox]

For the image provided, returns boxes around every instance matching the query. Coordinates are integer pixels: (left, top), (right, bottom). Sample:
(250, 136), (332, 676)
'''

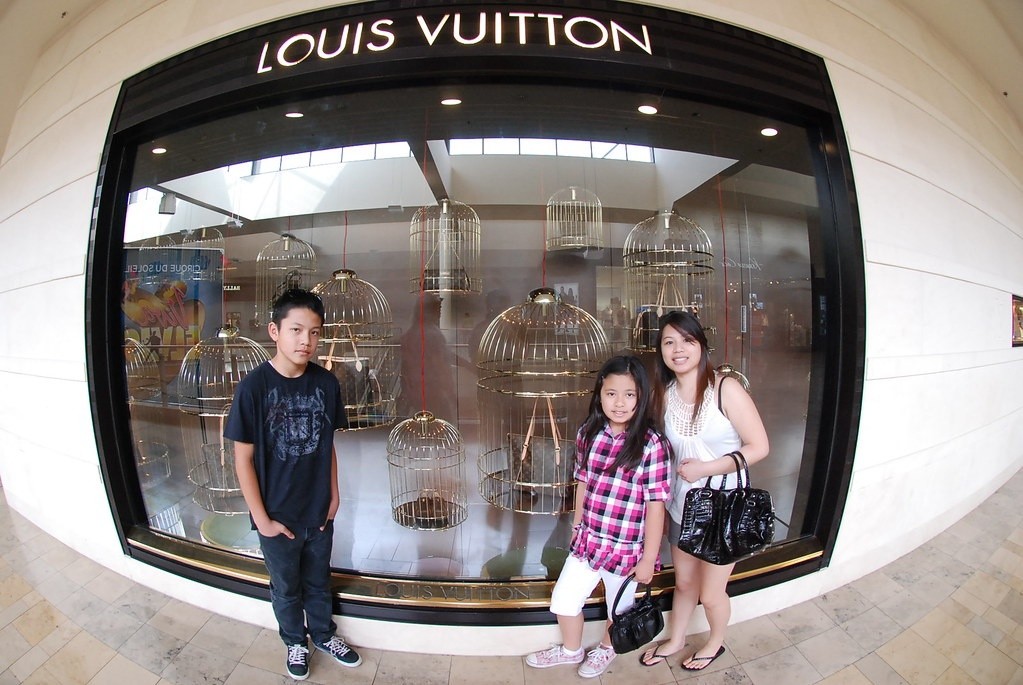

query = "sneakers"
(525, 642), (585, 668)
(286, 642), (309, 680)
(311, 634), (362, 667)
(577, 642), (617, 678)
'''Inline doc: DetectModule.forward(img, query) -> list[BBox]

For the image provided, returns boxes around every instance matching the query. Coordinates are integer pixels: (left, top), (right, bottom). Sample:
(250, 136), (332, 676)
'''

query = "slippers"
(639, 639), (668, 666)
(681, 646), (725, 672)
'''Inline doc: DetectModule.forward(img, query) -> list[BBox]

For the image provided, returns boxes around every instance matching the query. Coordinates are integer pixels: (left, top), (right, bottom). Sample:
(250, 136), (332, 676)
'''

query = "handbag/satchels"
(608, 572), (664, 654)
(678, 451), (775, 565)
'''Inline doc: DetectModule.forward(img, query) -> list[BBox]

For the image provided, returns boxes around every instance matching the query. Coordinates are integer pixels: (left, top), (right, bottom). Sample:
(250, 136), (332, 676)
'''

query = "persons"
(468, 288), (539, 505)
(639, 310), (770, 671)
(400, 299), (472, 441)
(223, 292), (363, 680)
(523, 356), (676, 678)
